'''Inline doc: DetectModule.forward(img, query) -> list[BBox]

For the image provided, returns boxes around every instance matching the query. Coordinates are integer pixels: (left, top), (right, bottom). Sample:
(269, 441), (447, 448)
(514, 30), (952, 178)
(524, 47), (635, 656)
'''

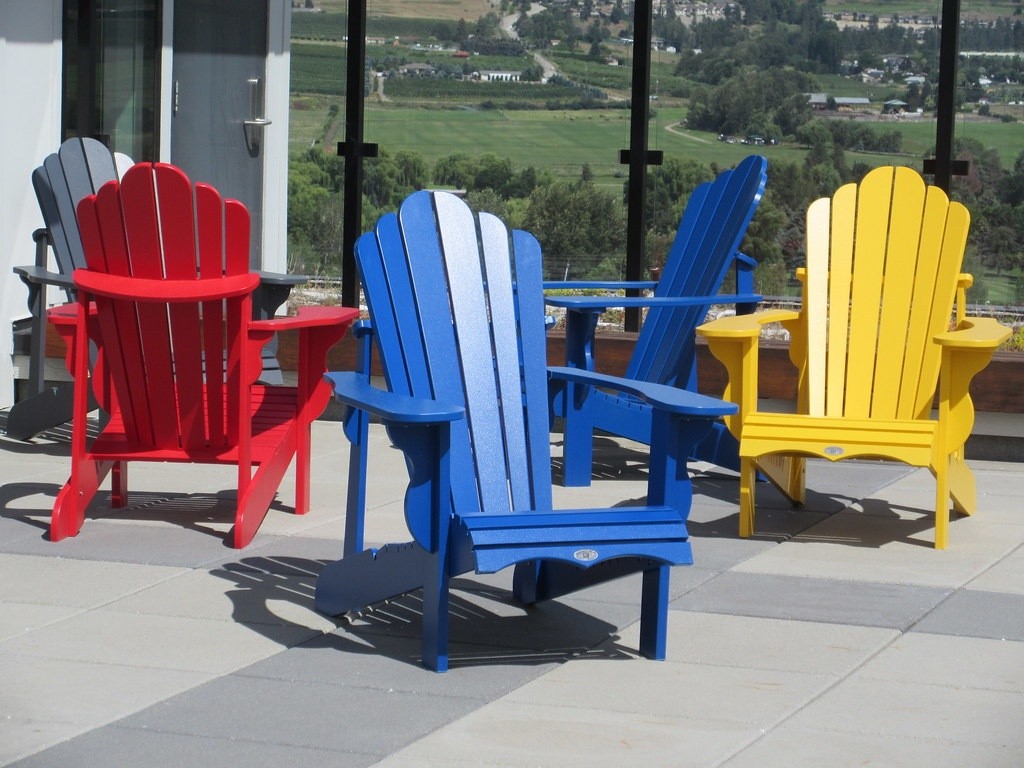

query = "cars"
(717, 132), (780, 146)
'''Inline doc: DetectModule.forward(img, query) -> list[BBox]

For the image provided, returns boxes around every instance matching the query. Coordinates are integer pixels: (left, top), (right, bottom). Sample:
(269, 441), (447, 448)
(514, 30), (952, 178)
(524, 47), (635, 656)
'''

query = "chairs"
(7, 135), (312, 442)
(481, 156), (769, 488)
(694, 164), (1015, 552)
(46, 162), (361, 549)
(320, 191), (740, 673)
